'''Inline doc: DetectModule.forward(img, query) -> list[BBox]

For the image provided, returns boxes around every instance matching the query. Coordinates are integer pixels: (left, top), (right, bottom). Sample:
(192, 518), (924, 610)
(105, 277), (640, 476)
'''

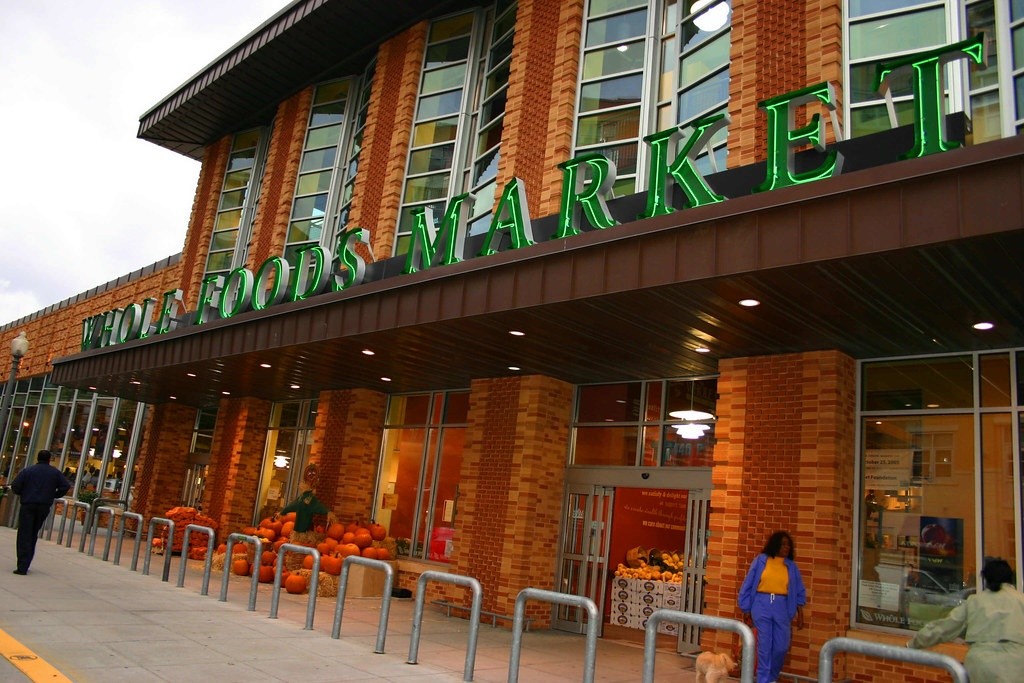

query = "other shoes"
(13, 569), (27, 575)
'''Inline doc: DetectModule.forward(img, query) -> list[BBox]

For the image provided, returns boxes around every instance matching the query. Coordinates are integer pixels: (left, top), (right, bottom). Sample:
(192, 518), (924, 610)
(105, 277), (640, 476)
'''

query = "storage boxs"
(347, 560), (399, 598)
(430, 524), (454, 563)
(246, 540), (273, 565)
(609, 546), (682, 637)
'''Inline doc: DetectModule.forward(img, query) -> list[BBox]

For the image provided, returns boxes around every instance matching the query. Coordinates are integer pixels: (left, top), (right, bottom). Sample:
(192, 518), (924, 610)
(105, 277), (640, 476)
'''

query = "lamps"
(670, 381), (714, 439)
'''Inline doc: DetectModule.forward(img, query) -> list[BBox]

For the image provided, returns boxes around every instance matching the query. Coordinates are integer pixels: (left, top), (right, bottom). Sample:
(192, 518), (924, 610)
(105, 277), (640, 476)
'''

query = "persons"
(273, 482), (337, 574)
(63, 466), (123, 483)
(11, 450), (70, 575)
(904, 560), (1024, 683)
(738, 532), (806, 683)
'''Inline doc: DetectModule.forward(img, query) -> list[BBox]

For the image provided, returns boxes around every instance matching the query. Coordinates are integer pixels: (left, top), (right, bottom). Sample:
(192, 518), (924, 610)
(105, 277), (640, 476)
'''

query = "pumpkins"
(152, 507), (389, 594)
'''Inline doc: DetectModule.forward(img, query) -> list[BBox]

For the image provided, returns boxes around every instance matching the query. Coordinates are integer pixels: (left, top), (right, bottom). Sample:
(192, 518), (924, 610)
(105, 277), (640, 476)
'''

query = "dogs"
(695, 651), (737, 683)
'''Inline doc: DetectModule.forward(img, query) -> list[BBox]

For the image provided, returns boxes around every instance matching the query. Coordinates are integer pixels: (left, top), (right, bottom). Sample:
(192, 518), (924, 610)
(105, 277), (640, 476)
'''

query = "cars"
(860, 563), (977, 639)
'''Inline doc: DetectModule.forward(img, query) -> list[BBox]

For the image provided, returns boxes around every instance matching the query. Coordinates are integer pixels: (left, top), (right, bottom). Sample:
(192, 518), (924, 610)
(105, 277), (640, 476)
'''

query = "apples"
(615, 552), (686, 583)
(922, 524), (957, 558)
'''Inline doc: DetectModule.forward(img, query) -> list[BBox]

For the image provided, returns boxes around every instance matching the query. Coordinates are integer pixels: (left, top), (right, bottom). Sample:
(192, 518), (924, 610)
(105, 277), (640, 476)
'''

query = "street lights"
(0, 330), (30, 438)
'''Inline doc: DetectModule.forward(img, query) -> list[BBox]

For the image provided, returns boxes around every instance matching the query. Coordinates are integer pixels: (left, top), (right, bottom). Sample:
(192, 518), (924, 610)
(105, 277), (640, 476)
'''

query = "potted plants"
(77, 490), (106, 527)
